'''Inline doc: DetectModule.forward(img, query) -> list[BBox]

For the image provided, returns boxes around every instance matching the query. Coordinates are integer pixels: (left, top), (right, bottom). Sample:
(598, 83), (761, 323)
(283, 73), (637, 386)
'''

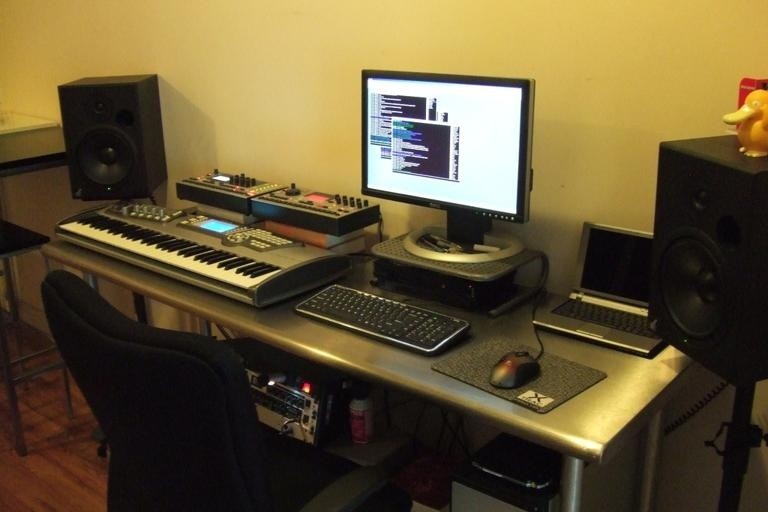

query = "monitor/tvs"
(361, 69), (536, 263)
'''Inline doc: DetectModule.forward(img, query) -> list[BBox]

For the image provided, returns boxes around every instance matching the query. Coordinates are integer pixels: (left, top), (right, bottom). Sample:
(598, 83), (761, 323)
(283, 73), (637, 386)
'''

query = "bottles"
(350, 385), (374, 444)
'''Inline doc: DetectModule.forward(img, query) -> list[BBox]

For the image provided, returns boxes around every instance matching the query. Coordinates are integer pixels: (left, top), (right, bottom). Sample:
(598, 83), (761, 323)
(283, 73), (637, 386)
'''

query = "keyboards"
(294, 283), (471, 358)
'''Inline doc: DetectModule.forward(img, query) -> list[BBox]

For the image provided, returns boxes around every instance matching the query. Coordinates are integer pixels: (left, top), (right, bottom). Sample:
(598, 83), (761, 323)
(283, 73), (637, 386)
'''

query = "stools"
(0, 217), (76, 426)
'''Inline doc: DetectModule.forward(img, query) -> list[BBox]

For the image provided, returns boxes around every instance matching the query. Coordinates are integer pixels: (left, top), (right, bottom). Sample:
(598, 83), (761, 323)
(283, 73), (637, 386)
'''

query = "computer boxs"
(449, 431), (562, 512)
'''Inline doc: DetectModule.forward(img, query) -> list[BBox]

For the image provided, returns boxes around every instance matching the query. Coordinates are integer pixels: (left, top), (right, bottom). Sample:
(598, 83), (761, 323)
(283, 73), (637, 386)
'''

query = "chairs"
(41, 269), (416, 512)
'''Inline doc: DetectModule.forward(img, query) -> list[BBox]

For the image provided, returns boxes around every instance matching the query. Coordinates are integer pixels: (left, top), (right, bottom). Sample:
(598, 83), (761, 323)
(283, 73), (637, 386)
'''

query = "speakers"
(649, 135), (768, 384)
(57, 74), (168, 201)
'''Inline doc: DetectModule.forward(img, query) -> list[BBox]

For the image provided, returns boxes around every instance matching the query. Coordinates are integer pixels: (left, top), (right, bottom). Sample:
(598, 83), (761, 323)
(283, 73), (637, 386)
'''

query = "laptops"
(532, 222), (678, 358)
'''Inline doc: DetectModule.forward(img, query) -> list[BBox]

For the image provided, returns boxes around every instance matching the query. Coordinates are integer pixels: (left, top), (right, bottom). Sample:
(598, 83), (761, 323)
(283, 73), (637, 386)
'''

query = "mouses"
(489, 351), (539, 388)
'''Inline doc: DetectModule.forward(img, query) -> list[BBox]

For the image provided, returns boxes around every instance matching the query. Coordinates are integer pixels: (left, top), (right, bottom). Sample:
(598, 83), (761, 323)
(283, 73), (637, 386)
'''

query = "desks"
(0, 110), (150, 460)
(39, 199), (697, 512)
